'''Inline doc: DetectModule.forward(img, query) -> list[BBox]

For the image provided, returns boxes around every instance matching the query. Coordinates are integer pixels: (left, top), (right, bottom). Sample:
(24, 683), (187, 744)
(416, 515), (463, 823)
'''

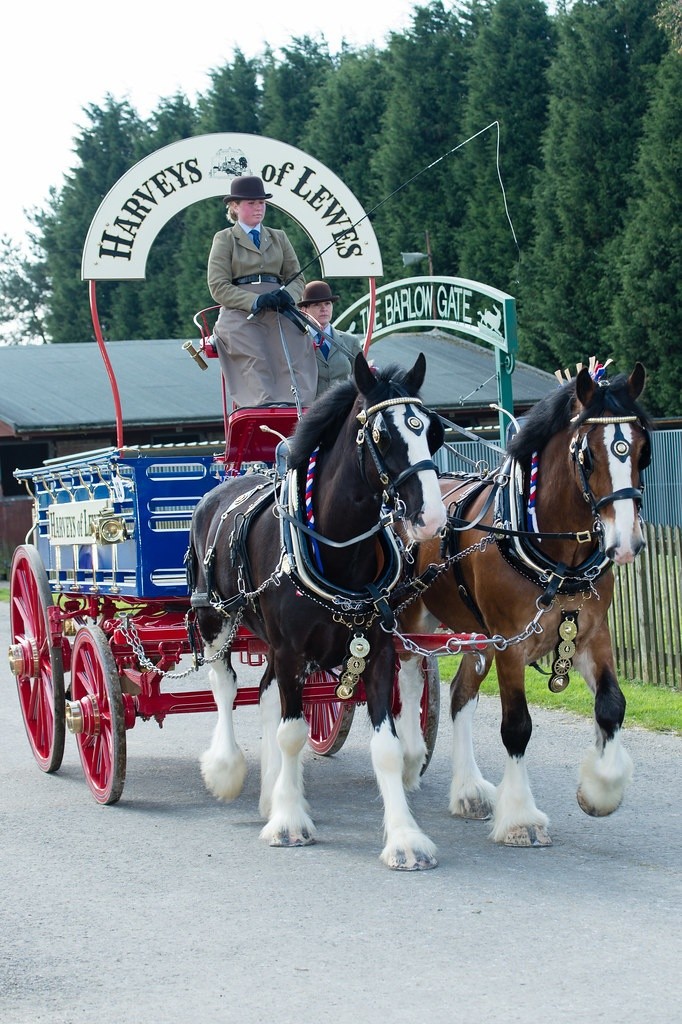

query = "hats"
(296, 281), (339, 308)
(223, 176), (273, 203)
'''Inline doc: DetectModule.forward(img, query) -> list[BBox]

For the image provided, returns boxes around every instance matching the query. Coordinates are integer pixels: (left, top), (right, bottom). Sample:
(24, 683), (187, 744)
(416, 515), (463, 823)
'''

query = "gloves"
(257, 289), (295, 312)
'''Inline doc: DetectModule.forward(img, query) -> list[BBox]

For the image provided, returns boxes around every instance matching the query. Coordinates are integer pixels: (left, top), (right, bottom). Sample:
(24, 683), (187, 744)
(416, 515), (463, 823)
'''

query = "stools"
(193, 304), (325, 442)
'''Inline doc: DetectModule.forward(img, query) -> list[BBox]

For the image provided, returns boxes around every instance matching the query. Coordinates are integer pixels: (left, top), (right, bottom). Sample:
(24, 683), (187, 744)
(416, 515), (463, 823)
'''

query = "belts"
(231, 273), (281, 285)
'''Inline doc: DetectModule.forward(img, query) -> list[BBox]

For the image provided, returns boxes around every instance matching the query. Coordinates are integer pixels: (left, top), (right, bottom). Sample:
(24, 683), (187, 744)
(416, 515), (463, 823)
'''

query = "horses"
(388, 361), (656, 848)
(189, 352), (448, 872)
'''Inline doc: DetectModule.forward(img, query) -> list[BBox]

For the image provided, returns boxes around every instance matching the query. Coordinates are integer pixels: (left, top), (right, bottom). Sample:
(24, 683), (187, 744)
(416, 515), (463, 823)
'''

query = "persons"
(296, 281), (365, 406)
(206, 175), (318, 408)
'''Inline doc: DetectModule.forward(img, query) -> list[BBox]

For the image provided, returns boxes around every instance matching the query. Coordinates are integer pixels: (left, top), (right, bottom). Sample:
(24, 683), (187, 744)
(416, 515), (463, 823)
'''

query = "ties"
(316, 333), (330, 361)
(249, 229), (261, 250)
